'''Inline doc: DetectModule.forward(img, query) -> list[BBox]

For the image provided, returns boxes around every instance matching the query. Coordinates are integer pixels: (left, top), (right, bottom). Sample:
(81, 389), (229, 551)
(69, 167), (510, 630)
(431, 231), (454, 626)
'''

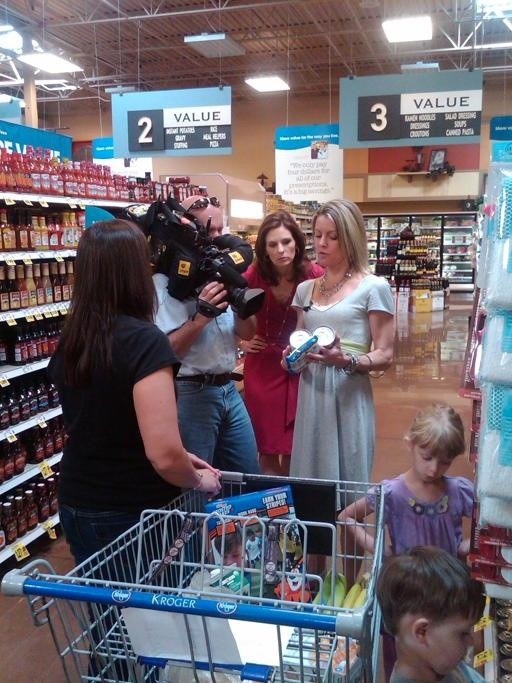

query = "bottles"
(0, 415), (68, 484)
(374, 230), (449, 292)
(0, 208), (80, 250)
(0, 261), (75, 311)
(1, 318), (65, 366)
(1, 464), (61, 550)
(0, 369), (59, 431)
(0, 145), (154, 203)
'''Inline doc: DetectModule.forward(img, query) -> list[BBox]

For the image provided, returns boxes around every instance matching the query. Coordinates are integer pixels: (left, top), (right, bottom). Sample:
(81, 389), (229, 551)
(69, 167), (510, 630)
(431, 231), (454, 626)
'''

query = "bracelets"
(193, 470), (203, 491)
(361, 351), (373, 372)
(345, 350), (359, 375)
(237, 337), (246, 347)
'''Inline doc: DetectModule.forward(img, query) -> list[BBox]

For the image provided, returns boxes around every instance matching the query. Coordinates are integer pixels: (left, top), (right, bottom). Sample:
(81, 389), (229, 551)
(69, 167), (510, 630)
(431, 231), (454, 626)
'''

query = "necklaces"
(319, 267), (354, 295)
(263, 272), (293, 345)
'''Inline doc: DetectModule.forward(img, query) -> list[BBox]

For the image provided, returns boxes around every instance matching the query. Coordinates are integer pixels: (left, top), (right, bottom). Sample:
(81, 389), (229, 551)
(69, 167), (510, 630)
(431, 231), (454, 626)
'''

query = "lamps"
(380, 14), (436, 44)
(15, 47), (86, 76)
(182, 31), (249, 60)
(241, 71), (291, 94)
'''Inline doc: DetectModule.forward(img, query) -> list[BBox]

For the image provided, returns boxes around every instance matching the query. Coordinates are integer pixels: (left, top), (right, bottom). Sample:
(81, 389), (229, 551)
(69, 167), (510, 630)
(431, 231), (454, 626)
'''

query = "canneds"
(289, 329), (312, 353)
(313, 325), (336, 349)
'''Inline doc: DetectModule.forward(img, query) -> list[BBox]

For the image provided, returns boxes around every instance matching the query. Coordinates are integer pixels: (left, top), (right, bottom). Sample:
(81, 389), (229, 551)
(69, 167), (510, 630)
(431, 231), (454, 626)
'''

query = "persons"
(150, 195), (262, 585)
(246, 522), (302, 569)
(213, 526), (242, 566)
(375, 544), (484, 683)
(281, 198), (395, 595)
(47, 219), (223, 683)
(336, 401), (476, 683)
(236, 208), (327, 478)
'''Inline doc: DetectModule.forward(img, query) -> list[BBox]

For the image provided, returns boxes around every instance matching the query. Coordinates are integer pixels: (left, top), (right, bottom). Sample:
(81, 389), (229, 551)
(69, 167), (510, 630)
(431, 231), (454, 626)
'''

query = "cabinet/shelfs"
(228, 213), (318, 261)
(362, 212), (478, 293)
(0, 191), (151, 564)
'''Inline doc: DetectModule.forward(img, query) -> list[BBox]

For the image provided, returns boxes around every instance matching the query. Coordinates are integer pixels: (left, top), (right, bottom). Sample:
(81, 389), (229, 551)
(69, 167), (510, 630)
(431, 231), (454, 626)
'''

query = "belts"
(179, 372), (245, 387)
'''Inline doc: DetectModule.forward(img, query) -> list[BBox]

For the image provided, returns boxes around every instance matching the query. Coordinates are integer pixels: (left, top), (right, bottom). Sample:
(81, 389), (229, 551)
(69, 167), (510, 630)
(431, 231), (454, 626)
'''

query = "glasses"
(186, 196), (220, 217)
